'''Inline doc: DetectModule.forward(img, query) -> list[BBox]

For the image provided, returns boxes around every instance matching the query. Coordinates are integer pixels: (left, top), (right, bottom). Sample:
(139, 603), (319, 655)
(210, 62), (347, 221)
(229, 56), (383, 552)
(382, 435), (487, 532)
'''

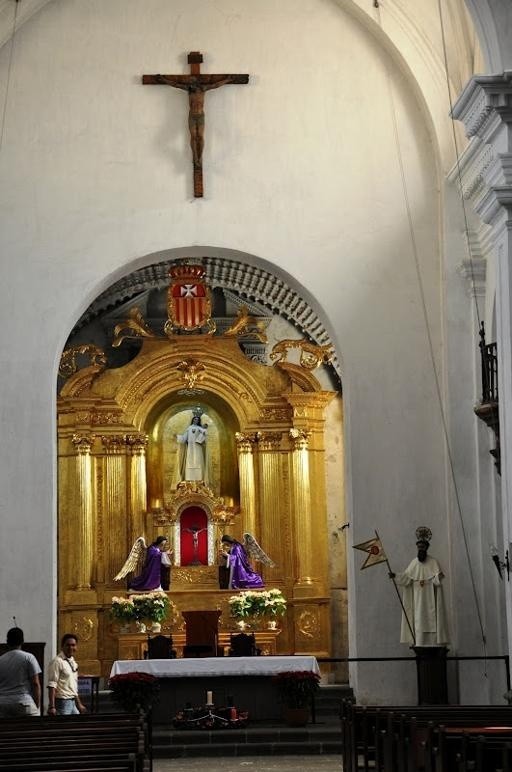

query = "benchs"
(0, 703), (153, 772)
(340, 700), (512, 771)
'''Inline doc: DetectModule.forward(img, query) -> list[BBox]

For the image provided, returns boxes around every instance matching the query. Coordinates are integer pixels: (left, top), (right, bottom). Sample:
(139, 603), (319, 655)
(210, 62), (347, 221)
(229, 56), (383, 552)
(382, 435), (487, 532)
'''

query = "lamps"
(487, 544), (510, 582)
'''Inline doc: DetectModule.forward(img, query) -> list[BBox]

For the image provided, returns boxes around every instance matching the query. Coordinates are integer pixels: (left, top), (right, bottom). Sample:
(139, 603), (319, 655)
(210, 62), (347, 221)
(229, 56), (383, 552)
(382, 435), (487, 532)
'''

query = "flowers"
(108, 671), (159, 709)
(239, 590), (269, 620)
(104, 594), (134, 624)
(263, 589), (289, 618)
(228, 595), (251, 622)
(144, 591), (177, 623)
(129, 593), (150, 623)
(280, 668), (321, 708)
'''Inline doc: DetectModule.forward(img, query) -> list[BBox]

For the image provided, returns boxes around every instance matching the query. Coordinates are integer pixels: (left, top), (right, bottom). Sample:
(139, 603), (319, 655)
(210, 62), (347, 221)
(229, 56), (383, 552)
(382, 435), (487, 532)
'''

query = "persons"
(170, 416), (209, 482)
(47, 634), (88, 717)
(0, 626), (42, 718)
(182, 526), (209, 562)
(388, 538), (451, 650)
(218, 533), (276, 589)
(113, 535), (174, 593)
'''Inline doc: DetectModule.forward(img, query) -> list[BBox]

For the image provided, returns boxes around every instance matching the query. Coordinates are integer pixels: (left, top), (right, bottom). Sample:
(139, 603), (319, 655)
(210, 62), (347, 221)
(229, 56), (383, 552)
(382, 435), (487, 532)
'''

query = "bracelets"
(48, 706), (57, 710)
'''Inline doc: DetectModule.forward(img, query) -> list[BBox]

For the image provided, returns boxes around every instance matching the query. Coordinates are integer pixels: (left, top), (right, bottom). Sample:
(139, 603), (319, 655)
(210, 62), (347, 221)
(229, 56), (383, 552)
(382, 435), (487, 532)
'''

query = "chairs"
(144, 632), (177, 658)
(227, 631), (259, 656)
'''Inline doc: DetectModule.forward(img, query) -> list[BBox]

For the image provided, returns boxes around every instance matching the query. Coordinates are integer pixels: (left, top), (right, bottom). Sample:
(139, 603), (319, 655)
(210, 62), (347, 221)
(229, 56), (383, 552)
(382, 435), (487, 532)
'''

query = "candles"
(206, 690), (215, 704)
(229, 705), (238, 719)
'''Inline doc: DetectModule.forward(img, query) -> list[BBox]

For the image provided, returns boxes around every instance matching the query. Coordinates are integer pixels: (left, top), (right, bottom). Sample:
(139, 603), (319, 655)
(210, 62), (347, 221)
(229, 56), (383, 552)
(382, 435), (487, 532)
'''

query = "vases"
(150, 619), (162, 633)
(252, 618), (264, 631)
(234, 618), (249, 631)
(118, 621), (131, 632)
(134, 620), (146, 631)
(266, 620), (279, 631)
(284, 702), (311, 727)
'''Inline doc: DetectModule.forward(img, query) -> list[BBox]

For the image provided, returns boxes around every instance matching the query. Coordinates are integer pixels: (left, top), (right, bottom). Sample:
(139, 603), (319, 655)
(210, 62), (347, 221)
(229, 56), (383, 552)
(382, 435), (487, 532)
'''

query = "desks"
(78, 673), (101, 712)
(111, 655), (323, 725)
